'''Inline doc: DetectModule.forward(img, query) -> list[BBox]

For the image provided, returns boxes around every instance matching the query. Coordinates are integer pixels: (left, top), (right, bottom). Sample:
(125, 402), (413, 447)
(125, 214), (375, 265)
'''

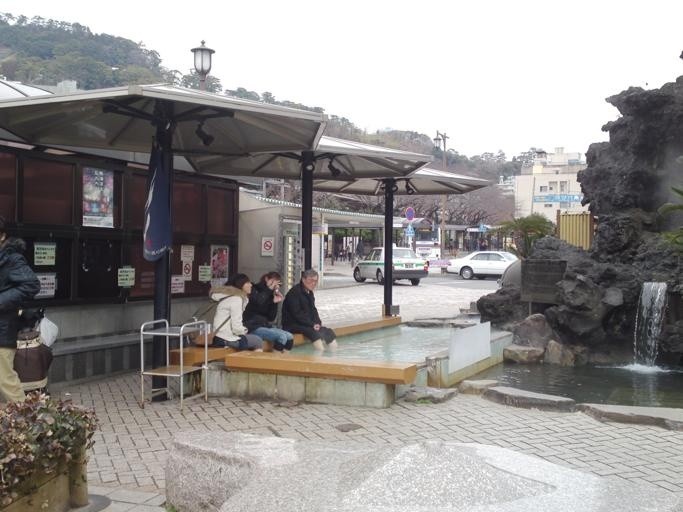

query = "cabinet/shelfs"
(140, 318), (209, 411)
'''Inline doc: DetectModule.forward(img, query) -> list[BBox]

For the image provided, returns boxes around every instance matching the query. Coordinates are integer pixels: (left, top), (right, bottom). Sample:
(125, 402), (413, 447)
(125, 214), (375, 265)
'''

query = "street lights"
(432, 130), (449, 259)
(191, 38), (214, 91)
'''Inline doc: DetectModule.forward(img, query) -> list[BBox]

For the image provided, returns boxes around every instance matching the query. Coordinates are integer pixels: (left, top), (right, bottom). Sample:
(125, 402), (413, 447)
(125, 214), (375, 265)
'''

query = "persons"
(206, 273), (263, 351)
(337, 241), (352, 261)
(0, 217), (41, 403)
(280, 268), (337, 348)
(244, 270), (294, 352)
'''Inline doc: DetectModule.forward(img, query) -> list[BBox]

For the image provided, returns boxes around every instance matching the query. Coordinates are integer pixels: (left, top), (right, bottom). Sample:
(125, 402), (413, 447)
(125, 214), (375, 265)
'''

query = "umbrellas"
(185, 131), (435, 318)
(312, 168), (491, 316)
(1, 78), (327, 401)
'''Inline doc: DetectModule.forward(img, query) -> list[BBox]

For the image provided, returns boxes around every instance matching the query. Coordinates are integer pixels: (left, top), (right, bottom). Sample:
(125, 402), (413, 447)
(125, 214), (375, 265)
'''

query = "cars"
(445, 250), (519, 281)
(352, 247), (428, 286)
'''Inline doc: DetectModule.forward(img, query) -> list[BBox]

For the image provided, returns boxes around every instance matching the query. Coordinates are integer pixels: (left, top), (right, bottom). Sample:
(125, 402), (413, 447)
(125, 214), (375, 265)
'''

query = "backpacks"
(189, 295), (244, 347)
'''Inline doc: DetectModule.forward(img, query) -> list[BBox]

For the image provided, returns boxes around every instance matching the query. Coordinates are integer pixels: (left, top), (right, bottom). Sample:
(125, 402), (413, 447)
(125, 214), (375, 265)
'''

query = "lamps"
(195, 118), (215, 146)
(405, 178), (414, 195)
(326, 156), (341, 177)
(297, 150), (315, 172)
